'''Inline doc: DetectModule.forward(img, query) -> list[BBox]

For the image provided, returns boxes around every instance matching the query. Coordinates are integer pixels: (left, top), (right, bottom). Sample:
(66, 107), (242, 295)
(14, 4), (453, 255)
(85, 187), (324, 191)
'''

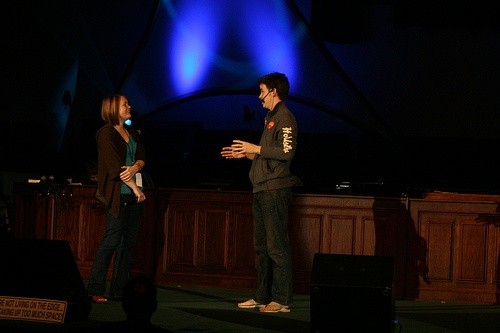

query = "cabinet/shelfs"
(10, 178), (500, 304)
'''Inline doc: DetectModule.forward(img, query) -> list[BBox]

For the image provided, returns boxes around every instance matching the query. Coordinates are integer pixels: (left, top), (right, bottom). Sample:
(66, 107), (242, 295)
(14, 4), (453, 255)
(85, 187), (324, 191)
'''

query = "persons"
(87, 94), (146, 303)
(220, 72), (298, 313)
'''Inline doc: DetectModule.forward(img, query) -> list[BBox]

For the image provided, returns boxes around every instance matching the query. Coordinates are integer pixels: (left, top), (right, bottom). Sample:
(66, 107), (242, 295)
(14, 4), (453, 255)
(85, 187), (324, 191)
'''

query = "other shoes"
(92, 295), (107, 304)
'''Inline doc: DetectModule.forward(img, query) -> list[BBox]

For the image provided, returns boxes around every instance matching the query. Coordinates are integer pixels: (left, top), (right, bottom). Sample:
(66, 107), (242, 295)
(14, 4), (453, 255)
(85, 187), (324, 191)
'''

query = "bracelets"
(134, 162), (141, 169)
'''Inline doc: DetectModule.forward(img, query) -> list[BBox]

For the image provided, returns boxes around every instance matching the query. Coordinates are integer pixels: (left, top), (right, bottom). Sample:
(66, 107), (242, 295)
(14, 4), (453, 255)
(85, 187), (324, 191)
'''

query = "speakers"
(310, 253), (395, 333)
(0, 238), (92, 322)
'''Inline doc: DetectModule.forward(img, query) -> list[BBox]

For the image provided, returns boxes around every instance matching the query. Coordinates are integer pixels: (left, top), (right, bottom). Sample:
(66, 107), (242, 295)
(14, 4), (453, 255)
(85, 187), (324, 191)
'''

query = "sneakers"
(238, 299), (266, 308)
(260, 302), (291, 312)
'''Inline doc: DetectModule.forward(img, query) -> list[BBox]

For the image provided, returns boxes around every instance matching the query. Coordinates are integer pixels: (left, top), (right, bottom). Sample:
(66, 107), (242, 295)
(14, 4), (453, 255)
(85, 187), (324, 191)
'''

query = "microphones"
(120, 196), (138, 208)
(261, 88), (273, 102)
